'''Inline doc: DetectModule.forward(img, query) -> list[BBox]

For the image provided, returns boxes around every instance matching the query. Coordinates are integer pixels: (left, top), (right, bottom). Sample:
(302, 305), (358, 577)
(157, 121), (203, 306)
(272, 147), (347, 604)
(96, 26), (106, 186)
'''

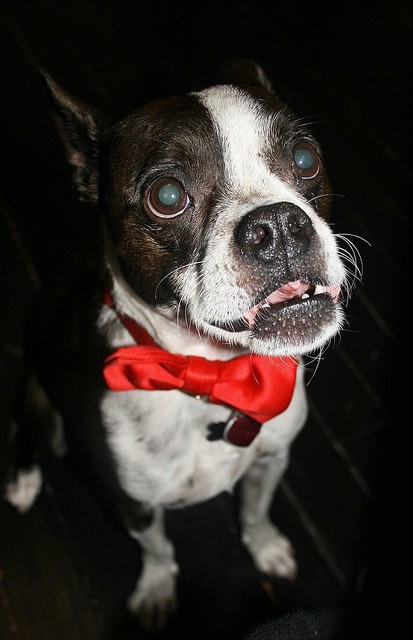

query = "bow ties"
(102, 294), (299, 426)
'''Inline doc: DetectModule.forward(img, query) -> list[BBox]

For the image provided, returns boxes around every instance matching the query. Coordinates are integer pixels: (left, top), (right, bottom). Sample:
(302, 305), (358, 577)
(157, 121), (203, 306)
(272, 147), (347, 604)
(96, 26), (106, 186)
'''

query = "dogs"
(5, 56), (372, 628)
